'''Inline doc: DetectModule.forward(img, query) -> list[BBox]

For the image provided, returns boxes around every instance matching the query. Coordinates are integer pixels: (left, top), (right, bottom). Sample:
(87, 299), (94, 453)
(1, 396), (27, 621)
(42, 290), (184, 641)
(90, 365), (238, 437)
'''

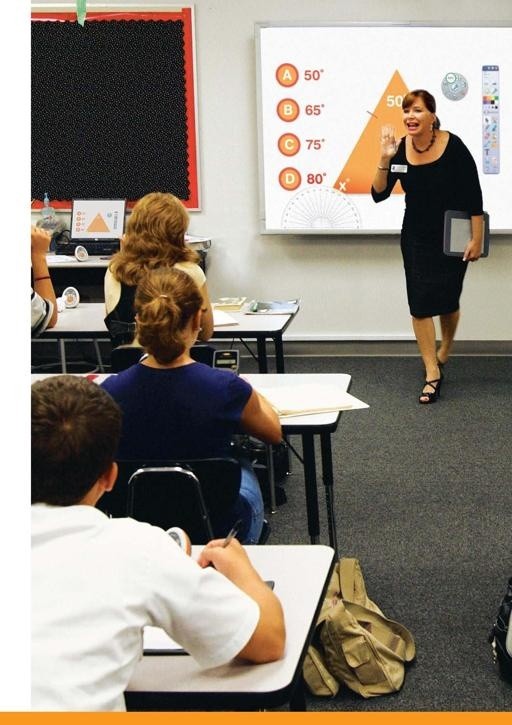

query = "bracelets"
(378, 166), (390, 170)
(34, 276), (50, 281)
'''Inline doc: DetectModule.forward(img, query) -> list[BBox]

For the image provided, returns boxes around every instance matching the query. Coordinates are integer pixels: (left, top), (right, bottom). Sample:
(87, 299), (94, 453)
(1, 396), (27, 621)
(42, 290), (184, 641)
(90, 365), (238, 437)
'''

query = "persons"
(30, 376), (285, 711)
(31, 228), (57, 337)
(371, 90), (484, 405)
(94, 267), (282, 544)
(104, 191), (217, 373)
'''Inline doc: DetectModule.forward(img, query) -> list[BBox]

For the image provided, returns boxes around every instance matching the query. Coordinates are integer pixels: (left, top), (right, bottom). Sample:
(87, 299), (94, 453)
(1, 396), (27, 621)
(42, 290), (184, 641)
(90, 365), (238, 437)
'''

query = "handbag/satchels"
(303, 558), (414, 701)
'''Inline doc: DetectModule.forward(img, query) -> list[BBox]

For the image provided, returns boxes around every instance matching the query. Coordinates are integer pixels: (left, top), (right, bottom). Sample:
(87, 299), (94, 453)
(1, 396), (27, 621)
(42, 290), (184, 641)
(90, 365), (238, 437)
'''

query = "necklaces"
(412, 129), (436, 153)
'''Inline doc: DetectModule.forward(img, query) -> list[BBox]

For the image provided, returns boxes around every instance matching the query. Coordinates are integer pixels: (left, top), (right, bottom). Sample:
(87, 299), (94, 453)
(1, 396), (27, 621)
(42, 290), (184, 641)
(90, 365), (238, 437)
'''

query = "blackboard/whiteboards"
(30, 3), (201, 213)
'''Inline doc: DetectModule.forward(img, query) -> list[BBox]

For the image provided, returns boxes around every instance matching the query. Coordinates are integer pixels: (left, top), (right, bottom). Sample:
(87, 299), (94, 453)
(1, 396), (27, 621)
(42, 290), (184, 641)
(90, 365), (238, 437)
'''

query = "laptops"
(55, 196), (127, 255)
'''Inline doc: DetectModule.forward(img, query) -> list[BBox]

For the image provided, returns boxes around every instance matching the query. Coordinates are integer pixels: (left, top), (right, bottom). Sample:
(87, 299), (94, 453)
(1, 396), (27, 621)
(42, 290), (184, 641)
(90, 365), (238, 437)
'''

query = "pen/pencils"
(209, 519), (243, 568)
(100, 257), (109, 260)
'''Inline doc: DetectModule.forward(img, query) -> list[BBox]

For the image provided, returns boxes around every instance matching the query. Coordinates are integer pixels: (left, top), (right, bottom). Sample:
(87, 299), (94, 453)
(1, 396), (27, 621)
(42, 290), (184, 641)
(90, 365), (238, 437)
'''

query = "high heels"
(419, 346), (448, 404)
